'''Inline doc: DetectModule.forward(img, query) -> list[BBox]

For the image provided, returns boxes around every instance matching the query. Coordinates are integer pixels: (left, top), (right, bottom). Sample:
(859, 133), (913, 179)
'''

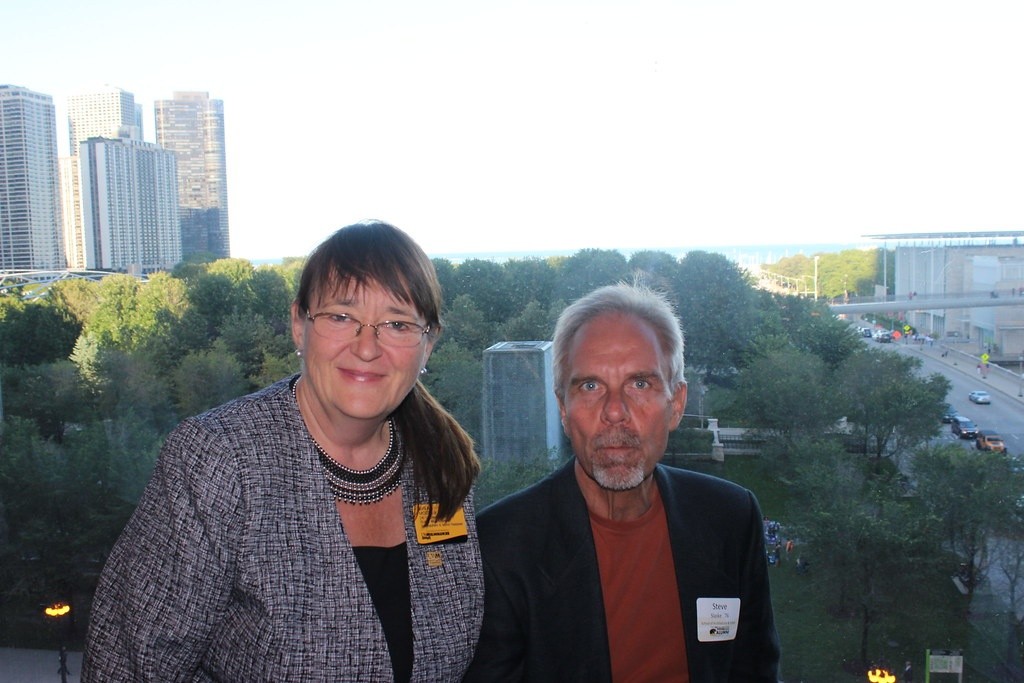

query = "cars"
(976, 430), (1005, 453)
(968, 391), (991, 404)
(951, 416), (979, 439)
(942, 406), (959, 423)
(862, 328), (890, 343)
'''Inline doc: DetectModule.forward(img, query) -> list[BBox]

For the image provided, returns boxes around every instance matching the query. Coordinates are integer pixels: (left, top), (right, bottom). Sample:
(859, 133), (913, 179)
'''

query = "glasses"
(305, 307), (432, 347)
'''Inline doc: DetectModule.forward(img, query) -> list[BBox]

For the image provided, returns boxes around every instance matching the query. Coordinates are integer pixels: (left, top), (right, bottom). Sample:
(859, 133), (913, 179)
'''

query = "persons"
(903, 330), (991, 374)
(82, 222), (485, 683)
(763, 516), (810, 574)
(901, 660), (912, 683)
(462, 284), (781, 683)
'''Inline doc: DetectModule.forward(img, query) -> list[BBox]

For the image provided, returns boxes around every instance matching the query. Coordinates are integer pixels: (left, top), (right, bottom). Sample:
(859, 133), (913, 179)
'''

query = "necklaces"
(286, 374), (402, 507)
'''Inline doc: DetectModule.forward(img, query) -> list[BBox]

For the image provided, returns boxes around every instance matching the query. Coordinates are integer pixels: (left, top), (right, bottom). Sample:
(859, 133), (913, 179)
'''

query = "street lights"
(45, 601), (70, 683)
